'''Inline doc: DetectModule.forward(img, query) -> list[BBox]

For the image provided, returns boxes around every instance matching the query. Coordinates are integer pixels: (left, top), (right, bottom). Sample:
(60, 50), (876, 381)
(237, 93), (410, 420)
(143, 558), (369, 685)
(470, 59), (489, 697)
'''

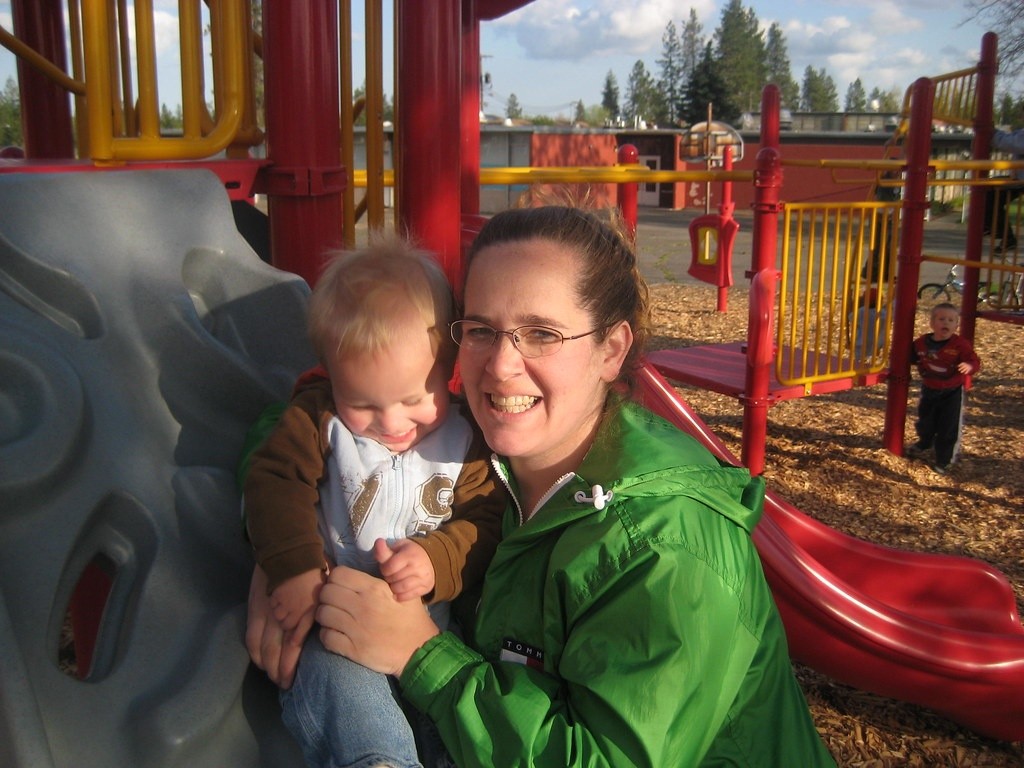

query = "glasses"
(448, 316), (619, 360)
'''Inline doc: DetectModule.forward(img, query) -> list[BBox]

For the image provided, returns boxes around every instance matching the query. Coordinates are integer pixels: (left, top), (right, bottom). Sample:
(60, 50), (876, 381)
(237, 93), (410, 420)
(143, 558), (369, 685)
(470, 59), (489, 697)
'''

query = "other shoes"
(909, 442), (931, 456)
(934, 459), (953, 473)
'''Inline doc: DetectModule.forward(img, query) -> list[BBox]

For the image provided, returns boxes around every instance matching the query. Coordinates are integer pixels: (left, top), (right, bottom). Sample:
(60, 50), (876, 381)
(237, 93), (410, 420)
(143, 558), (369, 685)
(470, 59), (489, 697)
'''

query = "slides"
(460, 212), (1024, 744)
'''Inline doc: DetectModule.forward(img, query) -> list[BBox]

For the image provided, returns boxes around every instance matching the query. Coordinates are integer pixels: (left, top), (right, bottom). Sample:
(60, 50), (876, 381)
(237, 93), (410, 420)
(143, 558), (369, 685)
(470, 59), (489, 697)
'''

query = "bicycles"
(918, 256), (1024, 317)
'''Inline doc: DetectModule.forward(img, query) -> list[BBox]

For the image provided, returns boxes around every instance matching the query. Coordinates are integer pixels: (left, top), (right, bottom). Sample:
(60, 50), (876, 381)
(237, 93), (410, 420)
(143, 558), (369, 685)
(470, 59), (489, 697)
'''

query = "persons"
(911, 303), (981, 473)
(242, 238), (508, 768)
(983, 103), (1024, 254)
(849, 288), (895, 358)
(861, 157), (901, 283)
(245, 205), (838, 768)
